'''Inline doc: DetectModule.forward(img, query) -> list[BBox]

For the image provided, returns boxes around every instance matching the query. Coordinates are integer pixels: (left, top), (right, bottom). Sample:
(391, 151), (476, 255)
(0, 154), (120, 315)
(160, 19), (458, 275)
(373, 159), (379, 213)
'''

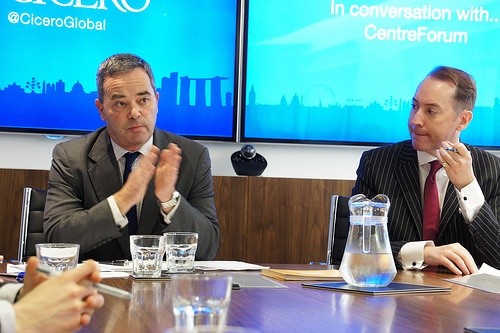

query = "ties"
(122, 152), (141, 235)
(422, 160), (443, 271)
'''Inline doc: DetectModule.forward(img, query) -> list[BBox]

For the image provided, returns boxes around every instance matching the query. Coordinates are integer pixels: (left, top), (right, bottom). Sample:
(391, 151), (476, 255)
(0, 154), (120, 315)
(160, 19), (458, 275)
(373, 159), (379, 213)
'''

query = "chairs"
(327, 194), (352, 265)
(17, 187), (48, 262)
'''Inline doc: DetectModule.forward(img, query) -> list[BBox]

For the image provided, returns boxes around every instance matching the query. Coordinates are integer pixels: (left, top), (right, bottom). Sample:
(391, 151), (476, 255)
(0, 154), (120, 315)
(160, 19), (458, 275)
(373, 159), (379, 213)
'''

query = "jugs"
(339, 194), (397, 287)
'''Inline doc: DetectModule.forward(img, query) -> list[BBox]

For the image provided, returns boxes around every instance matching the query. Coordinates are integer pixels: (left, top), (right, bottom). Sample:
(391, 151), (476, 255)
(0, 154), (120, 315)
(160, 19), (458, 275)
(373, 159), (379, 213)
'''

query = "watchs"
(160, 191), (180, 209)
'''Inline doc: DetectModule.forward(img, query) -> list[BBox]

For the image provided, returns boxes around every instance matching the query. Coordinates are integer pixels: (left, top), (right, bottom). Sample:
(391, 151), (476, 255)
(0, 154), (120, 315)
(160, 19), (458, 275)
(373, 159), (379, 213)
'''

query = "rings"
(442, 162), (448, 167)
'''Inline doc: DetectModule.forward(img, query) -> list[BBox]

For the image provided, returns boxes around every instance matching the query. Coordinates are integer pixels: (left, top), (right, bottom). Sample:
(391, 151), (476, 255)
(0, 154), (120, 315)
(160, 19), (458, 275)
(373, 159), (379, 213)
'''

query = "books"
(261, 269), (344, 281)
(301, 282), (452, 295)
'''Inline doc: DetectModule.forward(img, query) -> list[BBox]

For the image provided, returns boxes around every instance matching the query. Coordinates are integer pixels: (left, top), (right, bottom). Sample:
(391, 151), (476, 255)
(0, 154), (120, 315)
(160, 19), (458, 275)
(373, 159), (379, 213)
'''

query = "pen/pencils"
(36, 267), (133, 298)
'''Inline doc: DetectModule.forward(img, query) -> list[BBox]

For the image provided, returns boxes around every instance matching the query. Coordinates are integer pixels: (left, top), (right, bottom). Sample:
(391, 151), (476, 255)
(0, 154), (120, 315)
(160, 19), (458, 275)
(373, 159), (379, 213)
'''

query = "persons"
(352, 66), (500, 275)
(0, 256), (104, 333)
(43, 52), (220, 261)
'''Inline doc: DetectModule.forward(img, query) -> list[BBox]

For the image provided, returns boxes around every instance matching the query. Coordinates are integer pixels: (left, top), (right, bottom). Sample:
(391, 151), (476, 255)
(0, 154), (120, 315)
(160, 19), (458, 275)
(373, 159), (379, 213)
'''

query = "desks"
(0, 261), (500, 333)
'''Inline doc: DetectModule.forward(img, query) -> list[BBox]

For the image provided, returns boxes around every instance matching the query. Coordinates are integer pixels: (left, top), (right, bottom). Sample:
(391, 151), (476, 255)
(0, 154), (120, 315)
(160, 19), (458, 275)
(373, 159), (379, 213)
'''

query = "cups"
(130, 235), (165, 278)
(35, 243), (80, 273)
(172, 274), (232, 333)
(164, 232), (198, 273)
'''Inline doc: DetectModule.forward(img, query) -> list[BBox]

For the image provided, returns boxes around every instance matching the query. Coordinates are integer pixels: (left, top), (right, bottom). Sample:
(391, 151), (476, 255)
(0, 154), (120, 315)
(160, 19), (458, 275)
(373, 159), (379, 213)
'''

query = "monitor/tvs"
(0, 0), (242, 146)
(240, 0), (500, 151)
